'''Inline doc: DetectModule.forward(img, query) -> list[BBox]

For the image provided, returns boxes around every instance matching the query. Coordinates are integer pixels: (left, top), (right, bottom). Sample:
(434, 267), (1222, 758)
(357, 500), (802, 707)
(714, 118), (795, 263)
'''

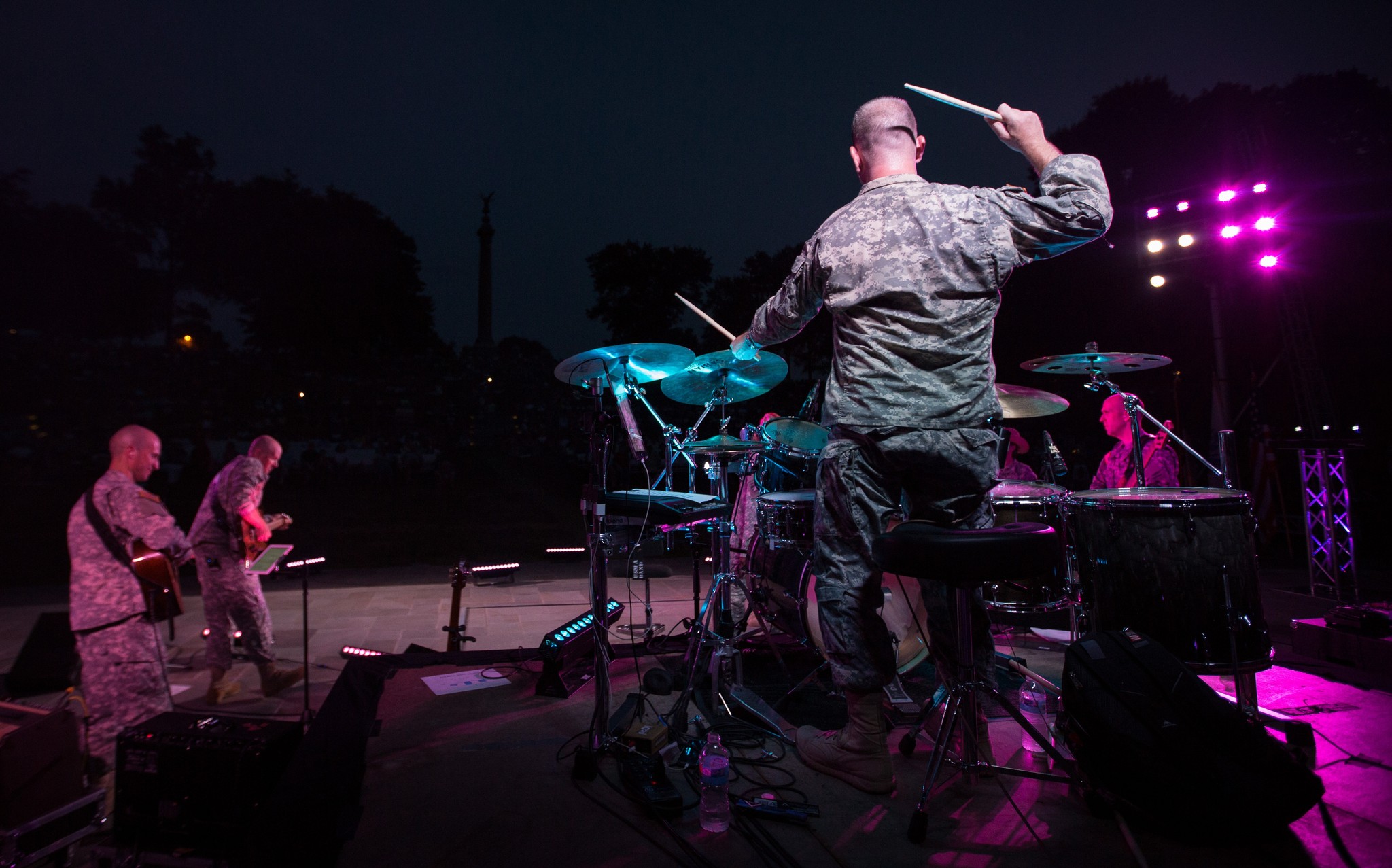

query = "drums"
(1058, 485), (1275, 674)
(746, 526), (934, 678)
(754, 416), (832, 496)
(756, 487), (819, 550)
(984, 478), (1080, 615)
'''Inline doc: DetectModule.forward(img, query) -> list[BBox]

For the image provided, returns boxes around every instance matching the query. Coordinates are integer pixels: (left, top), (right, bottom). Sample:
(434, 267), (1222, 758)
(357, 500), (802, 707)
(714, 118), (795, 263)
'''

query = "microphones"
(1043, 430), (1067, 477)
(798, 378), (821, 421)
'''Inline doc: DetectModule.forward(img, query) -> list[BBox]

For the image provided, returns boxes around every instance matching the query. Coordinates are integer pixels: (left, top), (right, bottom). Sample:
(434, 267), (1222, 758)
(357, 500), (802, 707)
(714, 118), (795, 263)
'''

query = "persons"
(997, 425), (1039, 481)
(1088, 391), (1183, 491)
(185, 435), (311, 705)
(723, 89), (1114, 798)
(65, 425), (198, 822)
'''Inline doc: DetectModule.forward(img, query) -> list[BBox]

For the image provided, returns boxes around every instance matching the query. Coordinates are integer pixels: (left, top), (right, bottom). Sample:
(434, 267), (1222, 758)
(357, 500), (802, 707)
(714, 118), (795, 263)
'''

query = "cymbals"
(660, 348), (790, 406)
(1019, 352), (1174, 376)
(554, 343), (696, 389)
(994, 383), (1070, 420)
(672, 434), (776, 455)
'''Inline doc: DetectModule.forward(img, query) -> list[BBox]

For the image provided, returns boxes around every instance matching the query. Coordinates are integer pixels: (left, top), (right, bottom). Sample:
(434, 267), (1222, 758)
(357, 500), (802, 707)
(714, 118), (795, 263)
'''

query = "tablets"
(607, 373), (649, 459)
(243, 544), (294, 575)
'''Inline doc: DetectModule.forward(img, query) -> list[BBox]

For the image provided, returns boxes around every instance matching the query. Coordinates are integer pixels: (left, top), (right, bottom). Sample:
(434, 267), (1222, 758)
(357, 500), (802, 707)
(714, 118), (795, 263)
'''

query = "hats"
(1001, 427), (1029, 454)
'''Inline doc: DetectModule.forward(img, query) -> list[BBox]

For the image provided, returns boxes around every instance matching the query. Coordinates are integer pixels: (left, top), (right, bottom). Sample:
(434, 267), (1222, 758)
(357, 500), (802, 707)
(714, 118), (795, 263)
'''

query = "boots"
(205, 668), (240, 704)
(922, 697), (996, 774)
(257, 661), (309, 698)
(794, 690), (894, 793)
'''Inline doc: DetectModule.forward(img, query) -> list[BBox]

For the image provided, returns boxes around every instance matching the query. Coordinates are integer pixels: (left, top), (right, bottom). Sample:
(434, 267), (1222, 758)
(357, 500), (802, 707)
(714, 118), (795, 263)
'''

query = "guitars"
(235, 512), (294, 561)
(132, 535), (183, 623)
